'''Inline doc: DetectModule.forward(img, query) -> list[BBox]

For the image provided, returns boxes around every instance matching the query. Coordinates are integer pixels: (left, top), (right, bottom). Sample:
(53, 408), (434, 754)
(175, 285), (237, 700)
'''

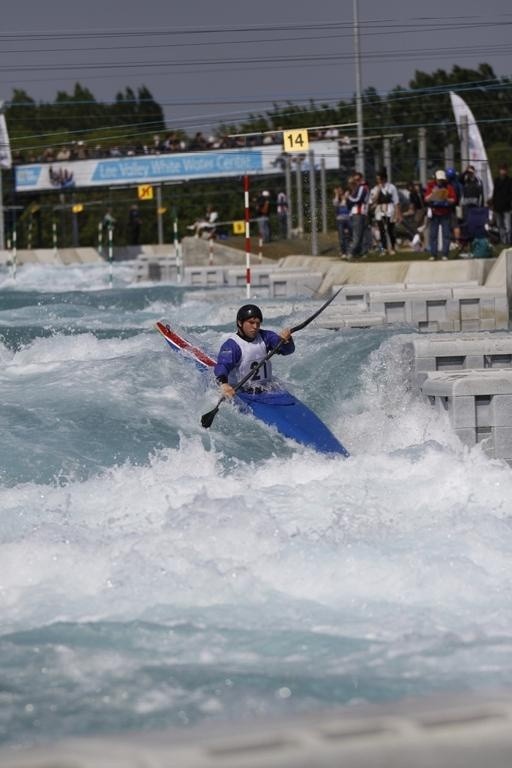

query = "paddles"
(202, 284), (345, 430)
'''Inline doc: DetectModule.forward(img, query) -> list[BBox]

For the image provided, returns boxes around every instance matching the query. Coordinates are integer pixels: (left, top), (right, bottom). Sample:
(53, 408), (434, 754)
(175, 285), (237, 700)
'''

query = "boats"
(153, 319), (351, 460)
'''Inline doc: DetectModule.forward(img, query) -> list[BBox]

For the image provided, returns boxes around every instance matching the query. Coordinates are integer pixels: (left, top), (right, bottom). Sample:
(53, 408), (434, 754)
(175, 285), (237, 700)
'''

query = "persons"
(214, 304), (296, 399)
(126, 203), (145, 245)
(276, 190), (288, 241)
(187, 202), (219, 239)
(330, 163), (511, 262)
(12, 125), (351, 164)
(254, 186), (273, 244)
(104, 206), (118, 243)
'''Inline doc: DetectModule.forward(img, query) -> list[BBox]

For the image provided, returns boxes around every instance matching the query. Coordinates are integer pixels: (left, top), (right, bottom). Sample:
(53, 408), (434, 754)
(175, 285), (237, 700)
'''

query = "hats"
(436, 170), (447, 180)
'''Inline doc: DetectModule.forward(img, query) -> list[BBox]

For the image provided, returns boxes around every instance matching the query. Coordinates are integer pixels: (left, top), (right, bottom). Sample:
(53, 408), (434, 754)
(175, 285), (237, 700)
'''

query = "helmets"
(237, 305), (262, 322)
